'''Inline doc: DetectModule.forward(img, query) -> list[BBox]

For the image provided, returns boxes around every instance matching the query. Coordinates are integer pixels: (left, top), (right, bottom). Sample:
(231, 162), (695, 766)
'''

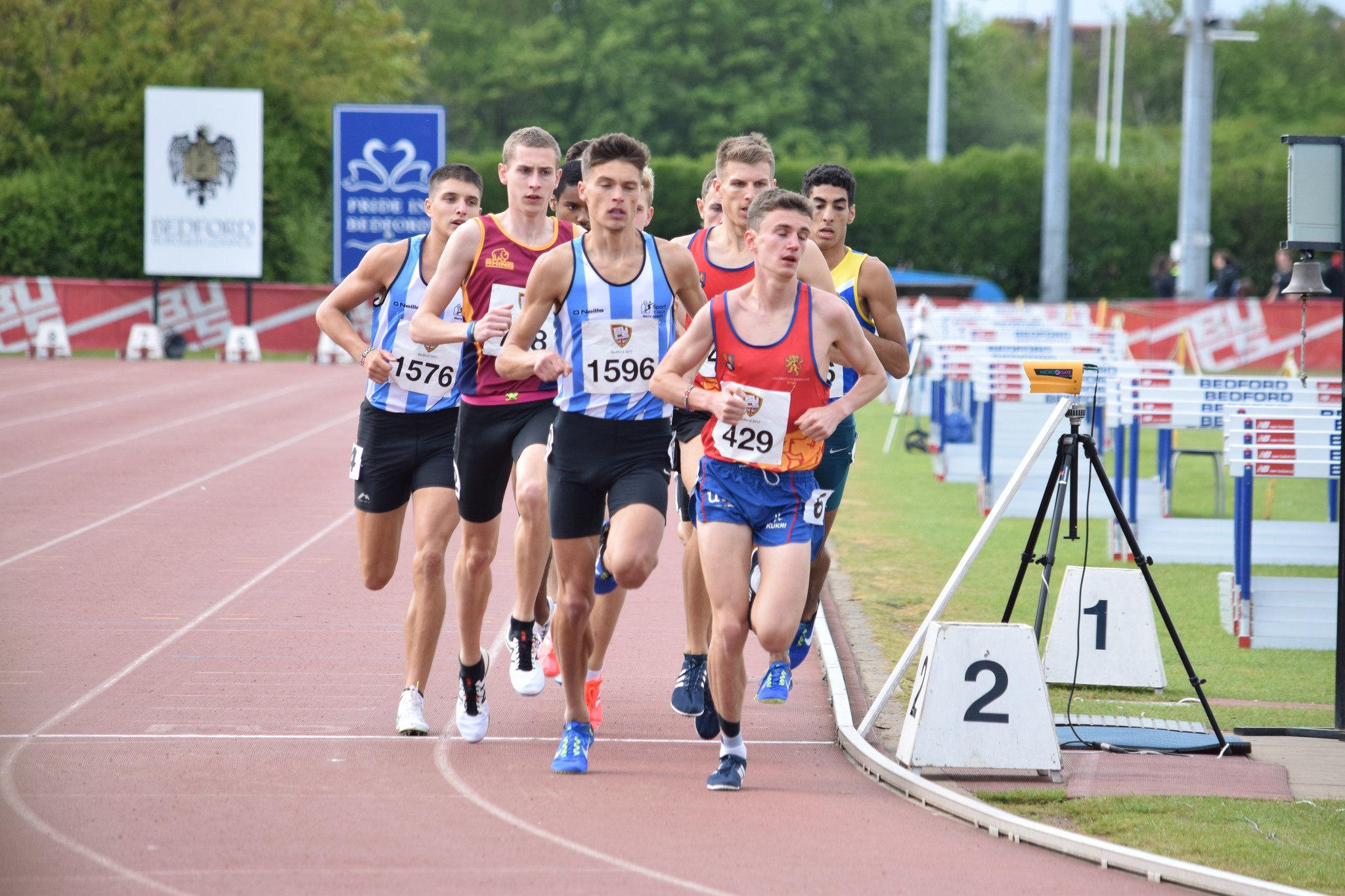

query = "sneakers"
(531, 596), (555, 653)
(583, 671), (603, 732)
(754, 660), (794, 704)
(593, 521), (618, 594)
(746, 547), (759, 629)
(455, 646), (490, 743)
(671, 651), (708, 717)
(789, 597), (821, 669)
(504, 624), (545, 696)
(706, 753), (748, 791)
(694, 677), (720, 740)
(395, 681), (430, 736)
(537, 624), (561, 677)
(551, 721), (595, 773)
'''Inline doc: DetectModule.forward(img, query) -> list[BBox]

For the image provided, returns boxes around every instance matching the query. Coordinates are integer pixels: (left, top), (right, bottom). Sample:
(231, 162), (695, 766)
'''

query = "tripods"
(1001, 411), (1233, 757)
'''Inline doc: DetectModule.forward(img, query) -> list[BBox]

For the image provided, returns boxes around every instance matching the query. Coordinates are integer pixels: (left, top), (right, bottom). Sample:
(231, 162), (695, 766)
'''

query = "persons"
(1149, 245), (1344, 298)
(493, 133), (709, 779)
(409, 126), (586, 744)
(534, 132), (912, 790)
(315, 165), (483, 736)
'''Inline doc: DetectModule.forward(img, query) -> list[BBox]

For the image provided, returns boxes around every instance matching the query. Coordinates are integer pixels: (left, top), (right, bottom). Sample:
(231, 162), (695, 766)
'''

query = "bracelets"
(467, 320), (477, 342)
(470, 321), (476, 342)
(683, 385), (695, 412)
(359, 347), (376, 367)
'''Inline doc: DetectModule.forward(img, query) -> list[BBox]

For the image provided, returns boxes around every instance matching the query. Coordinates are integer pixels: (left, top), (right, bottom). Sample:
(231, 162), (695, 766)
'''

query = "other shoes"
(554, 674), (562, 684)
